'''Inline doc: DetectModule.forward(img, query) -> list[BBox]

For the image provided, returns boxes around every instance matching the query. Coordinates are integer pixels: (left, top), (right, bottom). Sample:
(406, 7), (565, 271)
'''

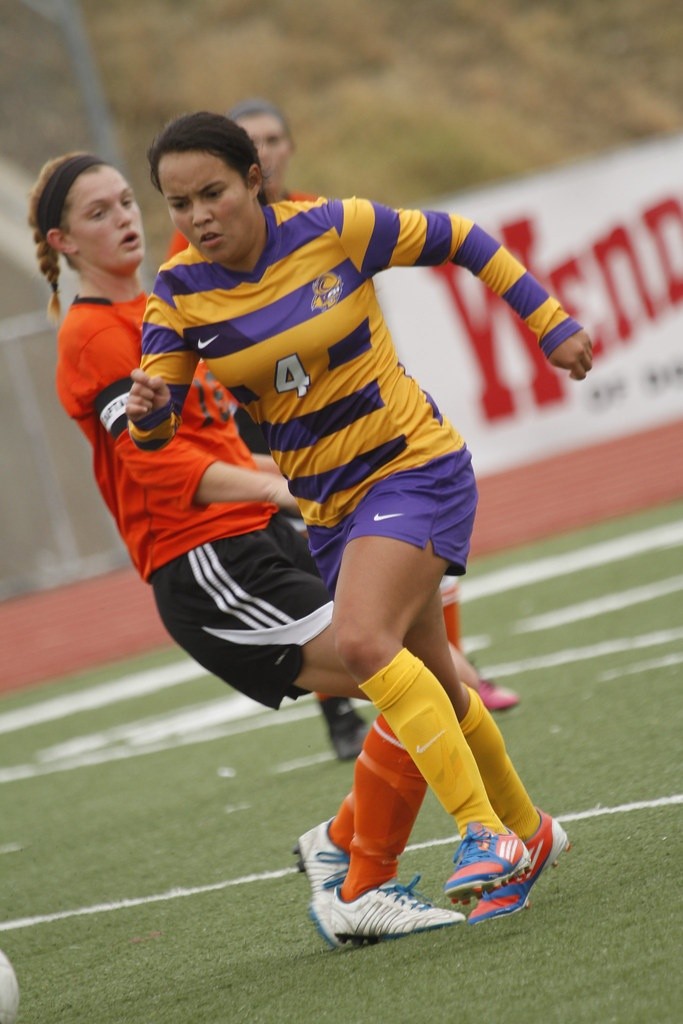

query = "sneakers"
(468, 807), (571, 925)
(330, 875), (465, 944)
(444, 822), (531, 905)
(292, 816), (354, 946)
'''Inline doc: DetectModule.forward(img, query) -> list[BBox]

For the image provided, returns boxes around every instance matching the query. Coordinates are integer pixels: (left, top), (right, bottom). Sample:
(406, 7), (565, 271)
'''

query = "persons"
(126, 107), (592, 925)
(162, 96), (519, 762)
(30, 150), (465, 949)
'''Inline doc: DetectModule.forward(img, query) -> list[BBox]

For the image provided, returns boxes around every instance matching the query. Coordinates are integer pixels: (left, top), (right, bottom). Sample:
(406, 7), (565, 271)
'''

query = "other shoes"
(476, 681), (519, 710)
(332, 726), (372, 758)
